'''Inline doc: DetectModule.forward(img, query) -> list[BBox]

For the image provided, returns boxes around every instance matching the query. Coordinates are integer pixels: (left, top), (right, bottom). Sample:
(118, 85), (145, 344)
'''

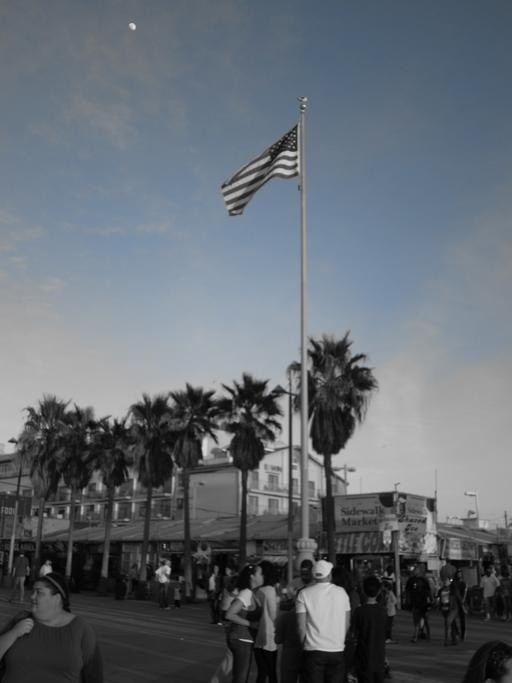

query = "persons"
(404, 554), (510, 682)
(203, 556), (397, 682)
(115, 559), (185, 608)
(0, 550), (105, 683)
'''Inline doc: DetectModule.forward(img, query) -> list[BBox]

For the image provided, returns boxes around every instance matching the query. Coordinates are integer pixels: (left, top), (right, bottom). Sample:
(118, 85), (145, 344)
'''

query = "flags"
(220, 124), (300, 217)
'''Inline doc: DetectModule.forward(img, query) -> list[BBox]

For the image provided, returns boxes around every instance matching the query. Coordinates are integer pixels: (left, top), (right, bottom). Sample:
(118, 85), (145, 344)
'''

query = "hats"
(310, 558), (334, 579)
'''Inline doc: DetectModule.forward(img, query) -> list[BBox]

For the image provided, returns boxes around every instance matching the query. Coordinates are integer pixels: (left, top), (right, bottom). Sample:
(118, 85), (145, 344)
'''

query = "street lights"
(332, 466), (340, 493)
(344, 464), (357, 495)
(464, 491), (478, 517)
(6, 436), (29, 574)
